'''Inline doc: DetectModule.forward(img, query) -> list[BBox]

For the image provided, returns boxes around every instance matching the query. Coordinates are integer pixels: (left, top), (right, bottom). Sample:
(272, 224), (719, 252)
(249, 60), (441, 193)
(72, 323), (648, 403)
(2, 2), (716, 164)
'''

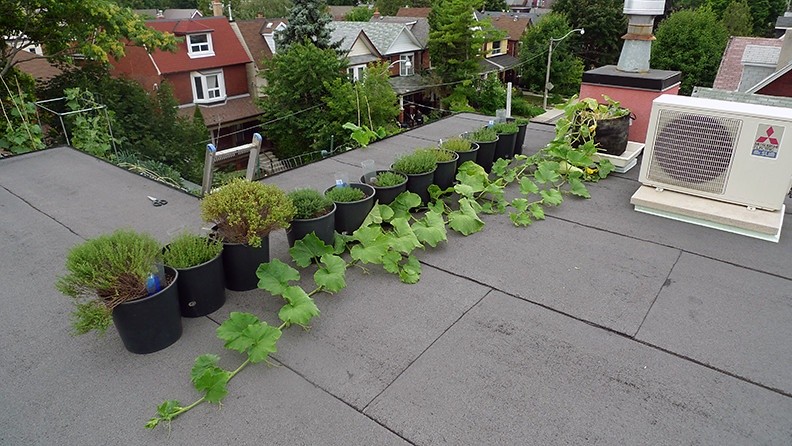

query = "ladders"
(202, 133), (262, 194)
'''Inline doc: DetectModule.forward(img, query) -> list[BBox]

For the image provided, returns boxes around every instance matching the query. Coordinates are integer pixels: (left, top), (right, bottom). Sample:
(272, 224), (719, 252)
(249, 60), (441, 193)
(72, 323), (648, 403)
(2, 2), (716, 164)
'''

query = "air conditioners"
(638, 94), (792, 211)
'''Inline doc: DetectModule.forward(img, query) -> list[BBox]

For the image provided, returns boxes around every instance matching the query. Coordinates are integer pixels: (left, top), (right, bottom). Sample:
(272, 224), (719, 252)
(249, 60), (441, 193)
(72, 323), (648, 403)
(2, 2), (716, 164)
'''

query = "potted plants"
(53, 90), (636, 441)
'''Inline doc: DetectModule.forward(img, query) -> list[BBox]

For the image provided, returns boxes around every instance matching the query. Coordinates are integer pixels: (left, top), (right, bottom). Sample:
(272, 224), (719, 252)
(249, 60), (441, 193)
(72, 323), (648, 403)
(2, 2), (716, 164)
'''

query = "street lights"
(542, 27), (585, 110)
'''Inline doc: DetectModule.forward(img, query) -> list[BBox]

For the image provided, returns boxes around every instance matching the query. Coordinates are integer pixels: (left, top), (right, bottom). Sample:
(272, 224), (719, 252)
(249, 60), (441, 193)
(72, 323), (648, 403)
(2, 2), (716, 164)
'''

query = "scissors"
(147, 196), (168, 207)
